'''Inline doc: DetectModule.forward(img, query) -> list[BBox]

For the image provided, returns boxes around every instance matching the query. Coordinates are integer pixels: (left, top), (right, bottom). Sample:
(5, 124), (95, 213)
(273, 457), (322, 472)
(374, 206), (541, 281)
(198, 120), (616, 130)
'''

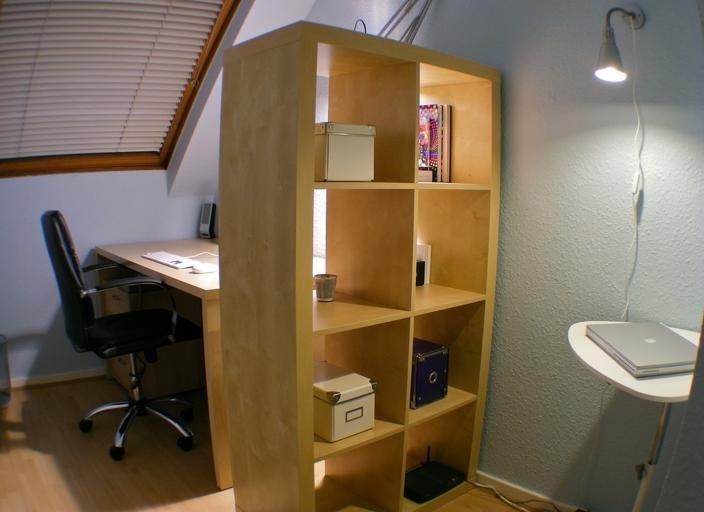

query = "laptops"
(586, 320), (699, 379)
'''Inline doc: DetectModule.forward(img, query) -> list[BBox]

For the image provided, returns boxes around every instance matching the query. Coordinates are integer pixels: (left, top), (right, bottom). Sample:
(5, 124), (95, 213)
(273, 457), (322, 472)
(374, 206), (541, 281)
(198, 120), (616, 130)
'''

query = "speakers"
(198, 202), (214, 238)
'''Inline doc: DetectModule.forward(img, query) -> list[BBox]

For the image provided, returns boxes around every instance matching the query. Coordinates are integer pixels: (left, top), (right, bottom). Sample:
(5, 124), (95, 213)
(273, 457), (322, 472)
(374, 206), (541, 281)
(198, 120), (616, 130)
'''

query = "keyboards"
(141, 249), (200, 270)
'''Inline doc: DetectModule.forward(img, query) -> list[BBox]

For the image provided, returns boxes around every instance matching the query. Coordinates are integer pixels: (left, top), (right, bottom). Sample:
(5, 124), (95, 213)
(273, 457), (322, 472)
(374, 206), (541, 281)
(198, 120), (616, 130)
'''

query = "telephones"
(199, 203), (215, 239)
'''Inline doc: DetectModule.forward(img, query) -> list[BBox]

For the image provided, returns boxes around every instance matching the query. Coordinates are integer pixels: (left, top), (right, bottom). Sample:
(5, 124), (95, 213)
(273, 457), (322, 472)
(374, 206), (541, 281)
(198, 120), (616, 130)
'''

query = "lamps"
(591, 3), (649, 86)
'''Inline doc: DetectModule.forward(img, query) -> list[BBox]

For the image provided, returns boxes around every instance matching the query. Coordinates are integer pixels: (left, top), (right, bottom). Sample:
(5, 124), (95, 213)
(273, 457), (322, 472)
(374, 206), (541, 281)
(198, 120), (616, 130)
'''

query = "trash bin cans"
(0, 333), (12, 406)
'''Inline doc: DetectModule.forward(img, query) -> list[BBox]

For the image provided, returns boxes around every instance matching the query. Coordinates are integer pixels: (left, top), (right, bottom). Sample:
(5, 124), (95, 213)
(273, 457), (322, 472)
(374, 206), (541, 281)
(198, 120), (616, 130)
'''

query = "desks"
(95, 233), (232, 495)
(568, 321), (701, 512)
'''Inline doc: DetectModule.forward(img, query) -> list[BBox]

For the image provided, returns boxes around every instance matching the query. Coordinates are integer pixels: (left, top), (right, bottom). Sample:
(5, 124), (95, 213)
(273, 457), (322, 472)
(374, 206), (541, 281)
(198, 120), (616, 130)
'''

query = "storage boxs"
(317, 118), (380, 185)
(311, 370), (381, 444)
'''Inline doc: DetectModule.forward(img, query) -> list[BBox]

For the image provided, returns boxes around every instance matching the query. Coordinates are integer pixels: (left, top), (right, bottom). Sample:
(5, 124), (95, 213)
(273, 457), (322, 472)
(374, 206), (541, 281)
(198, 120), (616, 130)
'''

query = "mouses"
(190, 263), (218, 275)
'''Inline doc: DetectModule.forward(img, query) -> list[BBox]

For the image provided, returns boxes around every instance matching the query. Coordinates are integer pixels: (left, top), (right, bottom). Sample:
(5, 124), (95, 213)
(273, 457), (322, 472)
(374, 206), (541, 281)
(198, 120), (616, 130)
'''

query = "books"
(417, 102), (449, 182)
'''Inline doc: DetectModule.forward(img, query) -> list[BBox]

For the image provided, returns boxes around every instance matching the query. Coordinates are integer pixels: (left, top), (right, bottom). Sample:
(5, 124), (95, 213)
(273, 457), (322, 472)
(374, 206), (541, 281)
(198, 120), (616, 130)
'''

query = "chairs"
(38, 208), (201, 462)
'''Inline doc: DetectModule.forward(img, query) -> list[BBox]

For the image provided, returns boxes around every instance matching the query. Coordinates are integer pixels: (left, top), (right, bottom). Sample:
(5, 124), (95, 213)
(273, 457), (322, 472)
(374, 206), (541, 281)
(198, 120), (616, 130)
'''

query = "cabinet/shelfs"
(215, 19), (505, 511)
